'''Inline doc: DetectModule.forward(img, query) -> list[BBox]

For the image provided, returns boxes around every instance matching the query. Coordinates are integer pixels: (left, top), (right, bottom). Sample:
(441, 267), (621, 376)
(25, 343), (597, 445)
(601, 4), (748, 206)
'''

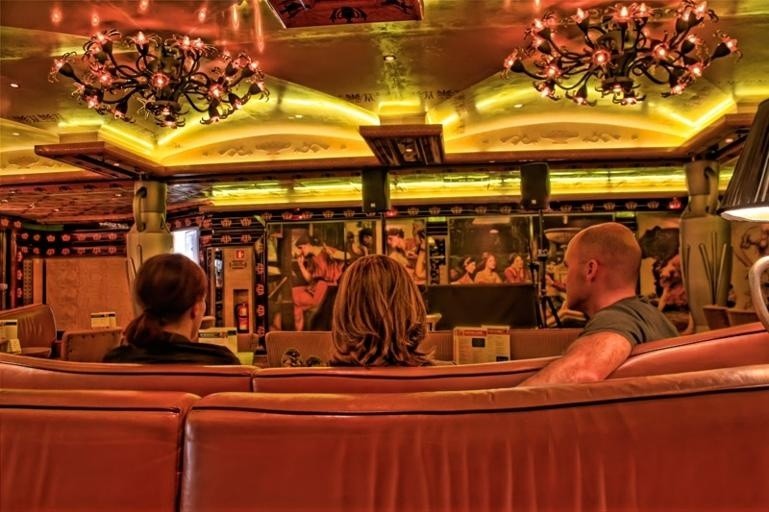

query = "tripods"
(533, 211), (561, 329)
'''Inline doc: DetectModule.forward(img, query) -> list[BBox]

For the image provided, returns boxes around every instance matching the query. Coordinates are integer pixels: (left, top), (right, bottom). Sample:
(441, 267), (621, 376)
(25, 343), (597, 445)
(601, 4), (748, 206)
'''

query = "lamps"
(47, 21), (270, 130)
(498, 0), (742, 108)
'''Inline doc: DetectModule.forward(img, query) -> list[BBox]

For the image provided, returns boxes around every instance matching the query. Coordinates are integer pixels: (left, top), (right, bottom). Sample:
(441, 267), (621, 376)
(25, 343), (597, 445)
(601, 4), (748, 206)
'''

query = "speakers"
(520, 162), (548, 211)
(360, 162), (389, 212)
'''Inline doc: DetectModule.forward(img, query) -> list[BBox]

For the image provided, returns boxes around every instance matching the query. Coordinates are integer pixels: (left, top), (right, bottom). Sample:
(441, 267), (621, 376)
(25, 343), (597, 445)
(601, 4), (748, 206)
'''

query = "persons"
(514, 222), (679, 388)
(385, 227), (419, 259)
(451, 252), (531, 283)
(348, 227), (373, 256)
(292, 235), (349, 332)
(325, 254), (440, 367)
(103, 254), (240, 365)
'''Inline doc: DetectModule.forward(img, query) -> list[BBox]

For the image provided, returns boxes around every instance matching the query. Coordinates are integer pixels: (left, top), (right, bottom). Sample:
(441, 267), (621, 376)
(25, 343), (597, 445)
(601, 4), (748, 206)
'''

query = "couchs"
(0, 305), (769, 510)
